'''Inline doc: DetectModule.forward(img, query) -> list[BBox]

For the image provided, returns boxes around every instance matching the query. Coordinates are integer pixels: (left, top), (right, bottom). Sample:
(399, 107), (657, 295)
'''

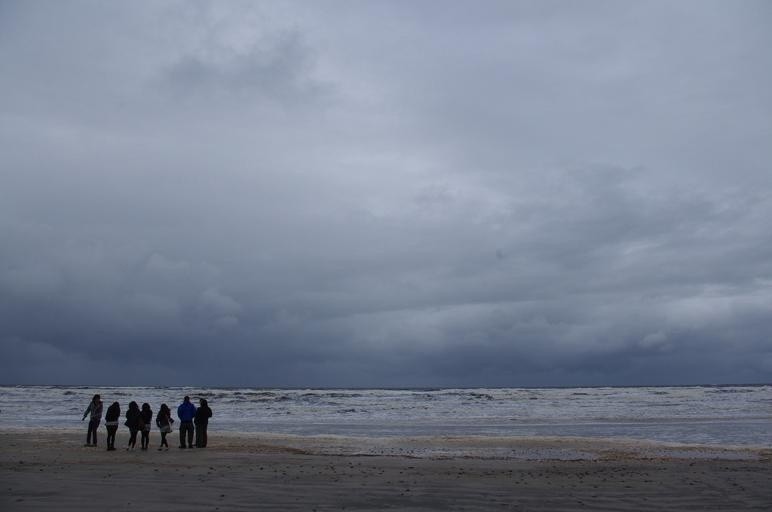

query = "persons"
(82, 394), (212, 450)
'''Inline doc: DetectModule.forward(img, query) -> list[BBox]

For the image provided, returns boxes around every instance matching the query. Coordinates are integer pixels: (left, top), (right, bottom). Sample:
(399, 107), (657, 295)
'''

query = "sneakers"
(85, 442), (208, 450)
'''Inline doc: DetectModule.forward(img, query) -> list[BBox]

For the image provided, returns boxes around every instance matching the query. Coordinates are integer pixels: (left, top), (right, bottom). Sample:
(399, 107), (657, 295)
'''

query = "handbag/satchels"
(169, 417), (174, 423)
